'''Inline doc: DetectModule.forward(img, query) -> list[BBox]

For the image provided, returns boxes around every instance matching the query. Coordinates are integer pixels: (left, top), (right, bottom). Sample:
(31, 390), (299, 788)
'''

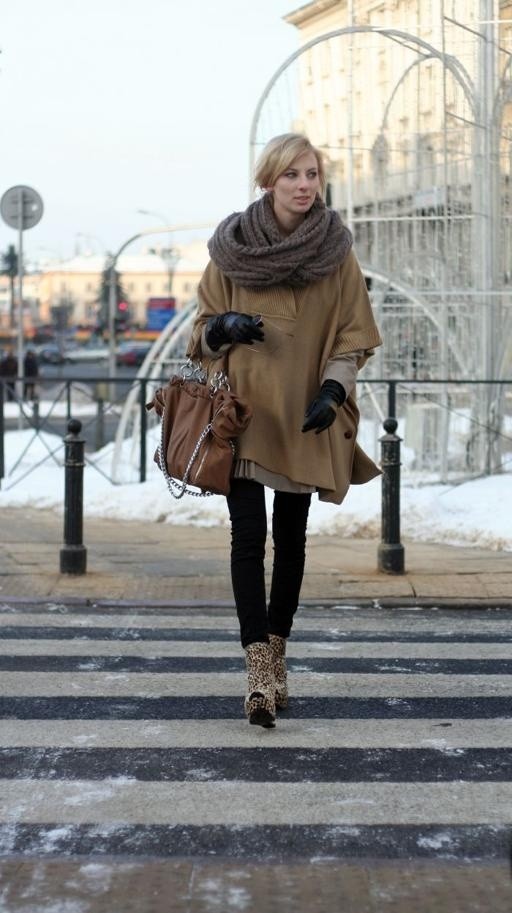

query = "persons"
(23, 349), (38, 400)
(183, 131), (384, 727)
(1, 350), (19, 401)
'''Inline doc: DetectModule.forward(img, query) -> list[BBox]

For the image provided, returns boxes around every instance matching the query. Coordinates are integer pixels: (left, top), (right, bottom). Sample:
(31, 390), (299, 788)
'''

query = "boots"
(243, 641), (278, 730)
(267, 632), (289, 709)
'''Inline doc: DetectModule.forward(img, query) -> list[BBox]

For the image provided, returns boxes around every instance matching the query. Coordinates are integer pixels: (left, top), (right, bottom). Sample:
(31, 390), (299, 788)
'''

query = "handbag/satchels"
(144, 328), (254, 500)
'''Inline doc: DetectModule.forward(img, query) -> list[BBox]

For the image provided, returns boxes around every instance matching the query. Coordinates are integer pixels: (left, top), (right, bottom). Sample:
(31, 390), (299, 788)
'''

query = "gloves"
(205, 310), (266, 347)
(300, 378), (347, 435)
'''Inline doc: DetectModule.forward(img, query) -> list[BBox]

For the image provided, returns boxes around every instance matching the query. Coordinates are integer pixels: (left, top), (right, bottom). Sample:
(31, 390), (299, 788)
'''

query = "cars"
(38, 338), (154, 367)
